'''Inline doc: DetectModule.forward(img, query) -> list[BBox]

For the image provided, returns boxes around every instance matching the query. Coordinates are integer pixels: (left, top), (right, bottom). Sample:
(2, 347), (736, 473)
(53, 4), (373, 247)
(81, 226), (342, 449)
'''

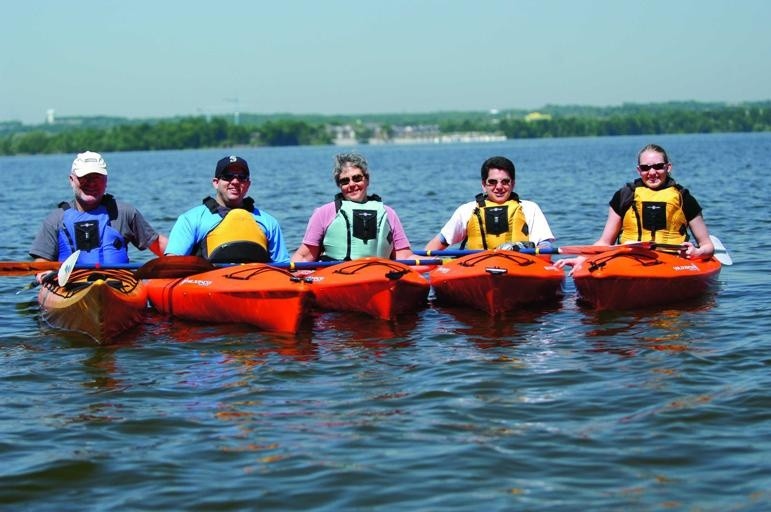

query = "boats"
(573, 244), (723, 313)
(430, 248), (566, 321)
(36, 266), (149, 345)
(298, 257), (431, 320)
(144, 262), (311, 336)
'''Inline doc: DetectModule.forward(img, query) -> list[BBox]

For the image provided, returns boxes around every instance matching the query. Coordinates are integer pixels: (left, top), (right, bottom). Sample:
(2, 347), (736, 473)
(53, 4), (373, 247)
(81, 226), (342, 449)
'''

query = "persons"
(289, 153), (441, 262)
(553, 144), (714, 277)
(163, 156), (288, 264)
(28, 151), (169, 280)
(421, 157), (554, 252)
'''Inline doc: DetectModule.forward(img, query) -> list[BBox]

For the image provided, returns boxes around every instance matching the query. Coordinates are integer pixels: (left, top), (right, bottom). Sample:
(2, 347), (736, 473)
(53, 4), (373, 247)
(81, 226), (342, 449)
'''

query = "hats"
(215, 156), (249, 178)
(71, 150), (108, 178)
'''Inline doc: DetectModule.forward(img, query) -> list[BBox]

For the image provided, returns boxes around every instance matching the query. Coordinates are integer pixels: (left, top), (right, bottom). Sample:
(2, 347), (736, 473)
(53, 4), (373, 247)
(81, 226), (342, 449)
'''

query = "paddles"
(0, 262), (143, 276)
(17, 249), (80, 295)
(413, 243), (649, 254)
(138, 256), (442, 275)
(654, 235), (731, 265)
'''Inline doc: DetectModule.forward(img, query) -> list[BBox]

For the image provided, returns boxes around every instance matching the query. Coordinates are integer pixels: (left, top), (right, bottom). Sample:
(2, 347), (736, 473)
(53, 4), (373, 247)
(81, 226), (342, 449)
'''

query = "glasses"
(339, 174), (365, 185)
(486, 178), (511, 186)
(639, 162), (666, 171)
(220, 172), (248, 180)
